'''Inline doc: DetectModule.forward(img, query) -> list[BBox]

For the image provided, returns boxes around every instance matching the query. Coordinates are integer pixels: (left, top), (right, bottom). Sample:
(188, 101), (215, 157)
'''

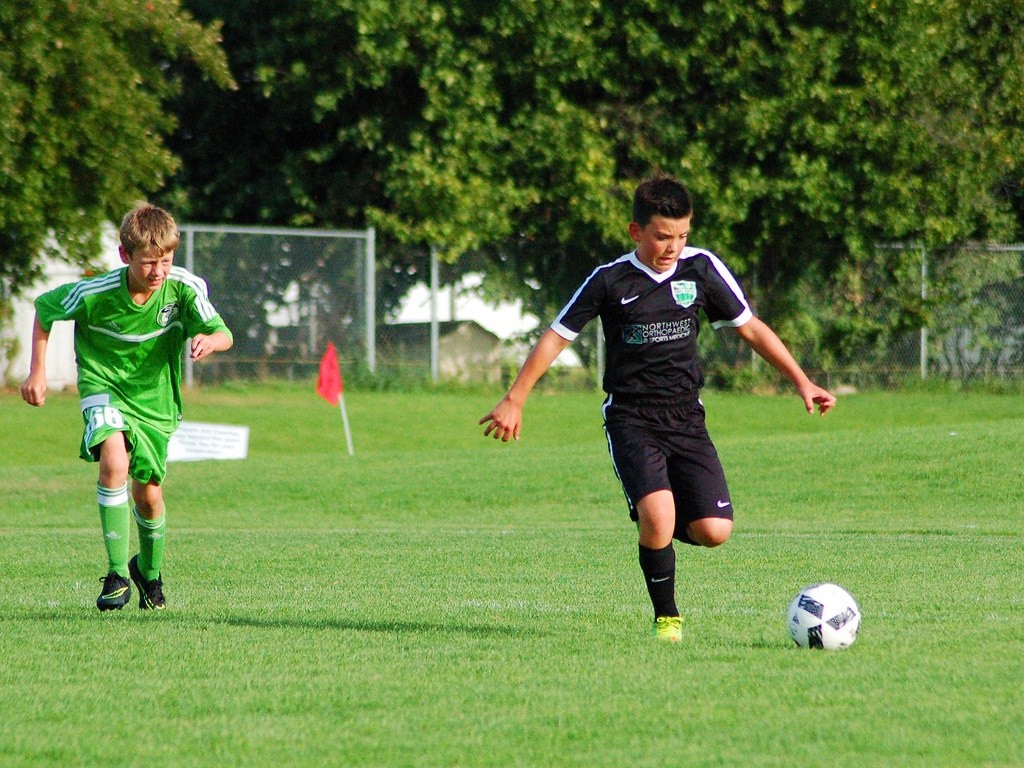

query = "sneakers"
(653, 617), (685, 642)
(129, 554), (166, 610)
(96, 571), (131, 611)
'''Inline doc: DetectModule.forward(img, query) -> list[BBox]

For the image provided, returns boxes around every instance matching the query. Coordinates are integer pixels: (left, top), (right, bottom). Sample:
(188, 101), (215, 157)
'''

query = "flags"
(316, 342), (343, 406)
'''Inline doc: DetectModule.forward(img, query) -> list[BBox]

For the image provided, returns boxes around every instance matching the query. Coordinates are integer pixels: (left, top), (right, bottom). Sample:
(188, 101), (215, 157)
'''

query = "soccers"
(784, 580), (863, 651)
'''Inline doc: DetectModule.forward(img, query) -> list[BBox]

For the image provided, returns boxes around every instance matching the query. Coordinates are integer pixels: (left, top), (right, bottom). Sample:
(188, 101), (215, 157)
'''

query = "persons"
(479, 179), (837, 642)
(21, 208), (234, 613)
